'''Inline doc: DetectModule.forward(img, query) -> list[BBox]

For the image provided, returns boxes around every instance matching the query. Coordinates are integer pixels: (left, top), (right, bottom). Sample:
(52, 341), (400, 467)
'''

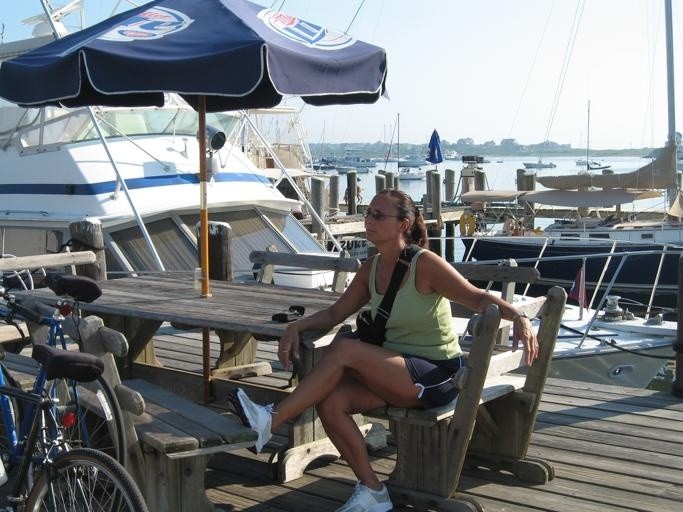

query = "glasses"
(364, 208), (398, 220)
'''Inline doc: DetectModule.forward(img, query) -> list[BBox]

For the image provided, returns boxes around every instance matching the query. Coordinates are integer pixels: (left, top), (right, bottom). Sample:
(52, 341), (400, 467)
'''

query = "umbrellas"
(0, 0), (388, 291)
(424, 128), (445, 170)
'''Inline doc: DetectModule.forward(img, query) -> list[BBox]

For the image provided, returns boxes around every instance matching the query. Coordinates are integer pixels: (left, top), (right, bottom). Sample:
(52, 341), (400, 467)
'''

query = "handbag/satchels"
(356, 310), (388, 346)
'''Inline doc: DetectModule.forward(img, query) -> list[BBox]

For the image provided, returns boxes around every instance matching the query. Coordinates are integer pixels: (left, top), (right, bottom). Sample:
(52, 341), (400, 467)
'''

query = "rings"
(280, 348), (289, 354)
(535, 345), (538, 349)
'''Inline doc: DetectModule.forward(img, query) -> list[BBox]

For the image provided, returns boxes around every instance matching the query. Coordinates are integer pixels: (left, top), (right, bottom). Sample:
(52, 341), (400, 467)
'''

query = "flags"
(568, 269), (588, 312)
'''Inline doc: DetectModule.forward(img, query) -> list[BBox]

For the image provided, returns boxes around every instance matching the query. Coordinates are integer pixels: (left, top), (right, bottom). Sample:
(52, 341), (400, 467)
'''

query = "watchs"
(510, 311), (529, 321)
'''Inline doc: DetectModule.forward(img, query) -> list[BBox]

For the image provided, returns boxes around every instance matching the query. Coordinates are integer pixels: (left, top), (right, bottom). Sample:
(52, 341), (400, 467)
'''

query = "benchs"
(67, 377), (257, 511)
(1, 246), (96, 290)
(249, 250), (363, 293)
(451, 262), (541, 358)
(5, 351), (42, 390)
(363, 286), (567, 511)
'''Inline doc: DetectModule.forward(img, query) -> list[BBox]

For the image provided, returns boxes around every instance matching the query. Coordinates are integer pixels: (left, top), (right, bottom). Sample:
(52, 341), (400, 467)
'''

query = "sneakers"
(331, 480), (394, 512)
(227, 388), (279, 454)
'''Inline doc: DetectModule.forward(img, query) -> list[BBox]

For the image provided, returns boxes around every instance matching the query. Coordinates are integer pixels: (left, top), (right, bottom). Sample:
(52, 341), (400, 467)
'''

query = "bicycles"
(0, 264), (150, 511)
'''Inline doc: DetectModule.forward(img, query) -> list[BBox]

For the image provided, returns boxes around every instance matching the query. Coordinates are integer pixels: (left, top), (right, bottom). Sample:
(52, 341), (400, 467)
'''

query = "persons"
(227, 187), (540, 512)
